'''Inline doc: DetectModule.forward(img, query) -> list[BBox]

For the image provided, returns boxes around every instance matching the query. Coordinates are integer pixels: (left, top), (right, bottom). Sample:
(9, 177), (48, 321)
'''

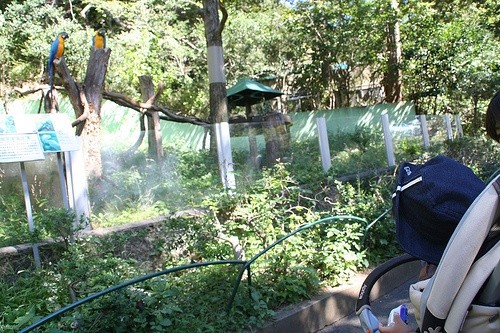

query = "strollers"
(353, 167), (500, 333)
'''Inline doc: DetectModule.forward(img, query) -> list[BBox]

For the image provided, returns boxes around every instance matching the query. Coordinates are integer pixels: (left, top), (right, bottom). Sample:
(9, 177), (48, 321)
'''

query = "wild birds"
(47, 31), (69, 103)
(92, 27), (107, 50)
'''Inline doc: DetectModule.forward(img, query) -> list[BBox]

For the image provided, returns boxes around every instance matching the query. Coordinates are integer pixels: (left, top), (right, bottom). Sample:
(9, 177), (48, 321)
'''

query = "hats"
(394, 155), (487, 266)
(486, 90), (500, 141)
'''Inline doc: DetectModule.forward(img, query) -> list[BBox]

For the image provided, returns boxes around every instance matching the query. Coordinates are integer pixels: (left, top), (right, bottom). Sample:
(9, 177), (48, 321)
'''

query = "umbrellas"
(226, 76), (285, 169)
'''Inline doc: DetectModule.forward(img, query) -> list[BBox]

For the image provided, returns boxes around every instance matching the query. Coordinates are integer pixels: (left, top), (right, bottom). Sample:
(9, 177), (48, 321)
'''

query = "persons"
(378, 90), (499, 333)
